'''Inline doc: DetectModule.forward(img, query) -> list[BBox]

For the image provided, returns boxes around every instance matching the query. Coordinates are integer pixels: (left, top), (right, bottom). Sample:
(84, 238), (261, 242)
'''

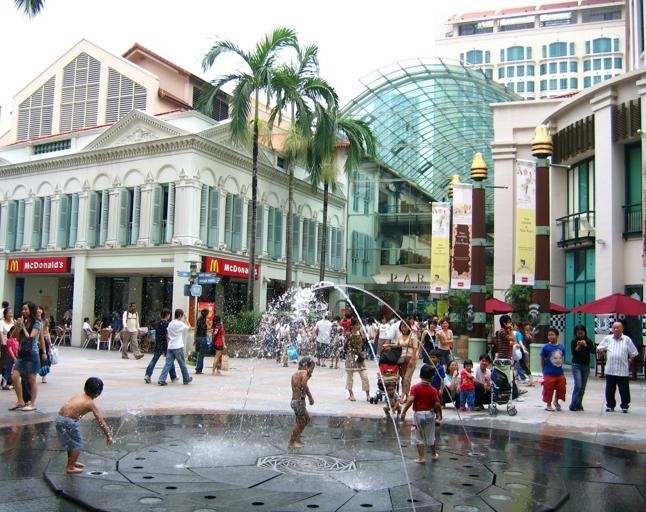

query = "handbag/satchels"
(380, 349), (400, 364)
(48, 344), (58, 364)
(38, 361), (49, 375)
(185, 346), (199, 366)
(287, 344), (298, 363)
(450, 343), (453, 349)
(354, 351), (366, 362)
(397, 357), (405, 364)
(221, 349), (229, 370)
(209, 343), (216, 355)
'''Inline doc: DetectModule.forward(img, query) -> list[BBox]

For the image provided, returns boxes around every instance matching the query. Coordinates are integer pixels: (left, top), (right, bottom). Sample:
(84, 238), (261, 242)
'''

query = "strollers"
(487, 353), (521, 417)
(367, 343), (403, 405)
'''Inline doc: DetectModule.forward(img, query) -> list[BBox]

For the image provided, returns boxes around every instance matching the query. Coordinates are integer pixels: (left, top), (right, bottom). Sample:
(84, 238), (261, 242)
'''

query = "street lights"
(183, 260), (202, 327)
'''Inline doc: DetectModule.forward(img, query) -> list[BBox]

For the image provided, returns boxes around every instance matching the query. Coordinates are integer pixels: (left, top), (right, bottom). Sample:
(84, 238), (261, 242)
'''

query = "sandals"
(19, 406), (36, 410)
(9, 402), (25, 410)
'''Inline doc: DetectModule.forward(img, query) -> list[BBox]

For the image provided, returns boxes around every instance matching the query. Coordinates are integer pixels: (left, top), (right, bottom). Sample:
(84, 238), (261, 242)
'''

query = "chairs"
(54, 323), (142, 351)
(625, 345), (645, 380)
(595, 344), (612, 378)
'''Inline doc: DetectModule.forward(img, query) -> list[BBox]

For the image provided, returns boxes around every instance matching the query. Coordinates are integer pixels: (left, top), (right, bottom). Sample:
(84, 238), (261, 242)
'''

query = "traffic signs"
(189, 285), (202, 297)
(176, 271), (190, 277)
(197, 278), (220, 284)
(197, 272), (216, 278)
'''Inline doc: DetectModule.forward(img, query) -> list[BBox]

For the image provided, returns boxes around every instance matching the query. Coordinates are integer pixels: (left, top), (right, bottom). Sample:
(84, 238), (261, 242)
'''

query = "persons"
(267, 312), (454, 404)
(399, 365), (444, 464)
(287, 356), (315, 449)
(595, 322), (638, 413)
(384, 350), (493, 423)
(540, 328), (567, 411)
(55, 375), (114, 474)
(193, 308), (227, 374)
(63, 309), (72, 336)
(0, 301), (57, 410)
(491, 317), (535, 401)
(83, 303), (144, 360)
(143, 308), (194, 385)
(569, 324), (595, 411)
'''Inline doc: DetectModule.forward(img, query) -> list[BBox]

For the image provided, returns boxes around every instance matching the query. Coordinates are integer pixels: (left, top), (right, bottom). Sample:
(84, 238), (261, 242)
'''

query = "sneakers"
(183, 377), (192, 384)
(553, 401), (560, 411)
(171, 375), (179, 383)
(606, 407), (614, 412)
(158, 379), (168, 385)
(544, 407), (554, 411)
(622, 409), (628, 413)
(144, 375), (150, 383)
(136, 353), (144, 359)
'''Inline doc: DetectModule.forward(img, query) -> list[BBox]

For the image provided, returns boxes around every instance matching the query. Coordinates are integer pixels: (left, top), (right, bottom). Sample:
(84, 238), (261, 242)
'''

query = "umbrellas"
(462, 297), (520, 337)
(570, 293), (645, 322)
